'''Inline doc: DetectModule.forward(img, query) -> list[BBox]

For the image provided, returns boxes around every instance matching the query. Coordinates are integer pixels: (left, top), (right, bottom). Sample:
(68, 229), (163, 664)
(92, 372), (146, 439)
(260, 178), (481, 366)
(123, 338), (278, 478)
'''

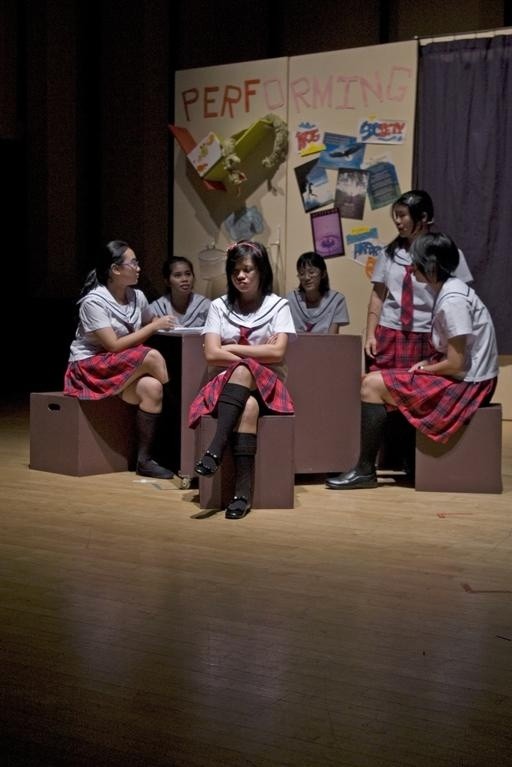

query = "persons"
(285, 250), (350, 334)
(63, 239), (180, 480)
(148, 254), (211, 327)
(323, 230), (500, 491)
(186, 239), (299, 519)
(364, 187), (478, 470)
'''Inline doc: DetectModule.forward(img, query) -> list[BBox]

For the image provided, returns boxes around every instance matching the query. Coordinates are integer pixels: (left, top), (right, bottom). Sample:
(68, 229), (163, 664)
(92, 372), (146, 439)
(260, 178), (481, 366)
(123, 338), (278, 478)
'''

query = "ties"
(237, 327), (253, 346)
(399, 264), (416, 328)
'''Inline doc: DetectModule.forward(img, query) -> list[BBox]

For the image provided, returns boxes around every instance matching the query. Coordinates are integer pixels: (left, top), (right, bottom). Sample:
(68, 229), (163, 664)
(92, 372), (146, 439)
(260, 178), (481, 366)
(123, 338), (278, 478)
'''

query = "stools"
(28, 393), (137, 479)
(194, 412), (295, 509)
(403, 402), (502, 493)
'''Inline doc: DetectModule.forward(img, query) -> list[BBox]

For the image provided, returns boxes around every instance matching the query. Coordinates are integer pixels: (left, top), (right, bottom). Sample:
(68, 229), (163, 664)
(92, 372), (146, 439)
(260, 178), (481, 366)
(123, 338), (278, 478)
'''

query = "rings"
(417, 365), (424, 369)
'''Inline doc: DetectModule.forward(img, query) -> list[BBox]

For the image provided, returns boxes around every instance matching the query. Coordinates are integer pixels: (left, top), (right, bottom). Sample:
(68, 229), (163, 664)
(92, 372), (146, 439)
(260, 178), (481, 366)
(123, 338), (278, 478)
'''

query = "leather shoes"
(224, 493), (254, 520)
(193, 447), (222, 479)
(323, 464), (379, 490)
(136, 458), (176, 480)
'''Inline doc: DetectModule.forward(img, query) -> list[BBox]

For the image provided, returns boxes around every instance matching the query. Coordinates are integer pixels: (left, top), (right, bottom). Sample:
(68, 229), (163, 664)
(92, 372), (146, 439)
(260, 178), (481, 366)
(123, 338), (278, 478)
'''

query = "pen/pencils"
(163, 303), (168, 315)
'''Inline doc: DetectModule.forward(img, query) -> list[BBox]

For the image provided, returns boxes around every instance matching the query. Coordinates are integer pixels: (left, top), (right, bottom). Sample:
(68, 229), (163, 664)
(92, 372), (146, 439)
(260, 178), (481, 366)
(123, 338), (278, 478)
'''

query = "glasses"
(115, 260), (139, 268)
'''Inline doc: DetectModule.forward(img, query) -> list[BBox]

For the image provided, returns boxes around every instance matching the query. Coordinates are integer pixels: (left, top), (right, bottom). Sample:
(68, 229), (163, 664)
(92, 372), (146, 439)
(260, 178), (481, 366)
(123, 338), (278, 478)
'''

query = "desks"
(142, 327), (364, 491)
